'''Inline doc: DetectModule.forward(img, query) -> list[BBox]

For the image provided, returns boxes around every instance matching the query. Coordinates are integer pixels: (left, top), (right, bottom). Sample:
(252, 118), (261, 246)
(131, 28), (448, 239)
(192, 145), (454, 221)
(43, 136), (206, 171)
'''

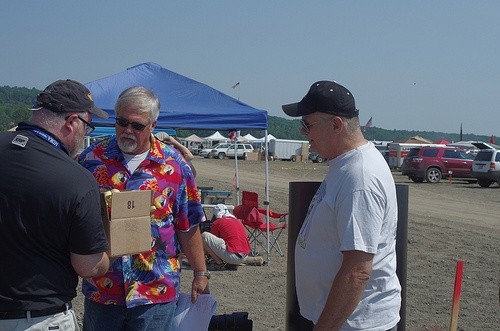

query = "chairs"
(233, 190), (289, 257)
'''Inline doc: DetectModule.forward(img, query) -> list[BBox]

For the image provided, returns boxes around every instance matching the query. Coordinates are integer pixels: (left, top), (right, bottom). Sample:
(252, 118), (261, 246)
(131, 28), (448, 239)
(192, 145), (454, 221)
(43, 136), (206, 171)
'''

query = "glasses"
(65, 114), (96, 136)
(116, 114), (151, 131)
(300, 116), (338, 133)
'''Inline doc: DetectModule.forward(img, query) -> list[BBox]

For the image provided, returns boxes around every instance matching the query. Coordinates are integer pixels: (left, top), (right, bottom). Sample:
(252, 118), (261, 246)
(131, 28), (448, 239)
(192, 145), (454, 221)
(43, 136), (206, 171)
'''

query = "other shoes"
(230, 264), (238, 271)
(209, 263), (226, 271)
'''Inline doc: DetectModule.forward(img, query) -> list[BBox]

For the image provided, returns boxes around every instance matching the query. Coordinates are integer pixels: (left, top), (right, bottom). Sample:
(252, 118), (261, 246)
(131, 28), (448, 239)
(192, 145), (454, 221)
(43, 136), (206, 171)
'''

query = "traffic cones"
(491, 133), (494, 144)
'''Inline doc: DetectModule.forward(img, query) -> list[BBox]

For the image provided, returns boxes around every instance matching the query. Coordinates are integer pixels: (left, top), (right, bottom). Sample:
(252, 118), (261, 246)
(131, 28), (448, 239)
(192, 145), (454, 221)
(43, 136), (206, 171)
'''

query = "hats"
(281, 80), (360, 118)
(36, 79), (110, 120)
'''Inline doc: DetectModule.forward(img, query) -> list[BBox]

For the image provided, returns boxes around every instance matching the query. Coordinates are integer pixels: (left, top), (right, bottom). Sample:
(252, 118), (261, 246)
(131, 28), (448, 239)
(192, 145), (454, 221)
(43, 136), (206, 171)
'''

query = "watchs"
(193, 271), (210, 279)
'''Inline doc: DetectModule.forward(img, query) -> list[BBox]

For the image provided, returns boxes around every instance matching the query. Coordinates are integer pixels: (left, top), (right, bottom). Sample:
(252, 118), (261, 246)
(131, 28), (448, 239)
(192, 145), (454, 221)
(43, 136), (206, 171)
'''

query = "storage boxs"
(99, 189), (155, 258)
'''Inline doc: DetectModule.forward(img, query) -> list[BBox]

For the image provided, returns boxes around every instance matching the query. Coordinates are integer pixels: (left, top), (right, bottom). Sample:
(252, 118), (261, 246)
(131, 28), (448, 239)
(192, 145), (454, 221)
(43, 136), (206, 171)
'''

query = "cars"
(469, 149), (500, 188)
(311, 152), (326, 163)
(199, 143), (254, 160)
(376, 144), (389, 155)
(401, 147), (477, 183)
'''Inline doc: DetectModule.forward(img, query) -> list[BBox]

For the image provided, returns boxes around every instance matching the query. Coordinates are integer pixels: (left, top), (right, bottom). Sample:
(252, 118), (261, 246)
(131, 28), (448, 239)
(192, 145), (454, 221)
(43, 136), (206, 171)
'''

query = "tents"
(33, 62), (270, 260)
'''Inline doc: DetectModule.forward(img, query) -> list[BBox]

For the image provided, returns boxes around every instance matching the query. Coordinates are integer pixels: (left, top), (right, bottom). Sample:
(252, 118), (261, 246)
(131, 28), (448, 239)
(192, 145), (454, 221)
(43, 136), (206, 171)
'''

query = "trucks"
(269, 139), (312, 161)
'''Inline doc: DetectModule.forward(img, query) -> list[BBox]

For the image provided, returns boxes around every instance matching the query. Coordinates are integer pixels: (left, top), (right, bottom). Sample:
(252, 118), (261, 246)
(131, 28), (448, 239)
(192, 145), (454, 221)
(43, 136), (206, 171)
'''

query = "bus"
(389, 143), (446, 168)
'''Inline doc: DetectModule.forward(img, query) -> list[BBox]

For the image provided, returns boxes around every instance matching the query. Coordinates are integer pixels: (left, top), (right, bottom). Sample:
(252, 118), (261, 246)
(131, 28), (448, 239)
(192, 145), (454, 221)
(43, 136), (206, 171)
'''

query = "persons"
(0, 79), (110, 331)
(164, 136), (197, 180)
(82, 87), (210, 331)
(282, 81), (401, 331)
(201, 204), (251, 270)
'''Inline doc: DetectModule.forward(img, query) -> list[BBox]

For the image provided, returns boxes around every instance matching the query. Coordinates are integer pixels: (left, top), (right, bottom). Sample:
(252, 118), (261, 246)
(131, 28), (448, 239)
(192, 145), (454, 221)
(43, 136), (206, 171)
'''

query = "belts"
(0, 303), (73, 320)
